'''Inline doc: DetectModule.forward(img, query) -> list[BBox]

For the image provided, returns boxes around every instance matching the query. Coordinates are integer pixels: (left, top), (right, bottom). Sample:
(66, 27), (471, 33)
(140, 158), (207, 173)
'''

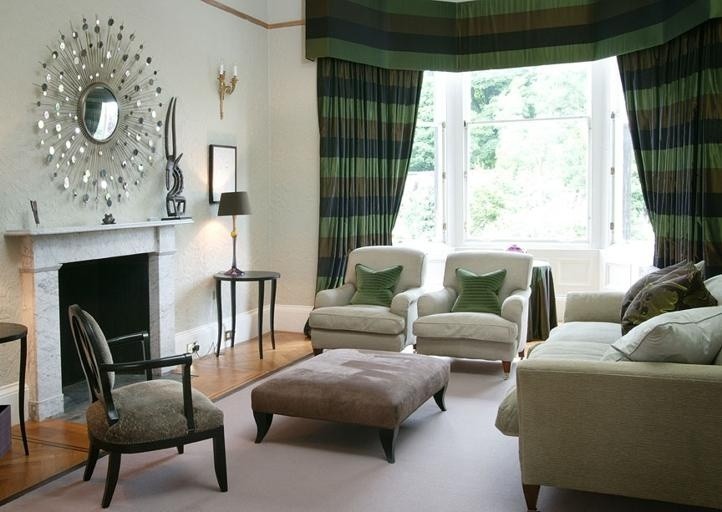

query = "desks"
(0, 321), (29, 456)
(212, 271), (280, 359)
(527, 260), (557, 342)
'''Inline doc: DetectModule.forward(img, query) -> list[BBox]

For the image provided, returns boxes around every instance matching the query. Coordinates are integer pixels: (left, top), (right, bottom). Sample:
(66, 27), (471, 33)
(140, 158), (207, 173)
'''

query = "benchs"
(250, 347), (451, 463)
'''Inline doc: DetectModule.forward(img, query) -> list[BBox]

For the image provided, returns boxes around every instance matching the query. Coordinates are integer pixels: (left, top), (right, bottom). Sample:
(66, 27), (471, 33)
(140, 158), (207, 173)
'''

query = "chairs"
(308, 246), (428, 353)
(411, 250), (533, 380)
(69, 304), (228, 508)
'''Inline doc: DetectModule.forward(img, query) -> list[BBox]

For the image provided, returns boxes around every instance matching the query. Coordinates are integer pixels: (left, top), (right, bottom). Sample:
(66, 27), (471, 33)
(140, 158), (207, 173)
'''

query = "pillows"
(598, 258), (722, 367)
(349, 263), (403, 307)
(450, 267), (507, 316)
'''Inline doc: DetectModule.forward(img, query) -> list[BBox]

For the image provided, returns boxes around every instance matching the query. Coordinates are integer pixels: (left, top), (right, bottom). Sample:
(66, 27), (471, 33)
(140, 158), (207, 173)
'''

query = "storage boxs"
(0, 405), (11, 458)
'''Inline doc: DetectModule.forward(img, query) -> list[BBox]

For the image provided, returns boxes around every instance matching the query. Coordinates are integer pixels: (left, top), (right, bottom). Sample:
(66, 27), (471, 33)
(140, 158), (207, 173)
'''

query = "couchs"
(495, 288), (722, 512)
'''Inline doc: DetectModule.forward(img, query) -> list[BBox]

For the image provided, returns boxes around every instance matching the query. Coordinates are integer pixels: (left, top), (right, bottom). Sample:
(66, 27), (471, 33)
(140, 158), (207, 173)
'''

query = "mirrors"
(78, 82), (121, 143)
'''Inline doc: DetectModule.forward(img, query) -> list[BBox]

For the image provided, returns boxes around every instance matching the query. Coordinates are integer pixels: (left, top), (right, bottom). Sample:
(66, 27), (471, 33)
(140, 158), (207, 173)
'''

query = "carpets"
(0, 340), (722, 512)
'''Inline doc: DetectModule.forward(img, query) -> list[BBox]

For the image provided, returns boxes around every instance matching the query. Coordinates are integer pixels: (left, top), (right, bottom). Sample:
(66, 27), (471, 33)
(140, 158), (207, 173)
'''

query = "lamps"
(217, 192), (253, 275)
(218, 64), (239, 120)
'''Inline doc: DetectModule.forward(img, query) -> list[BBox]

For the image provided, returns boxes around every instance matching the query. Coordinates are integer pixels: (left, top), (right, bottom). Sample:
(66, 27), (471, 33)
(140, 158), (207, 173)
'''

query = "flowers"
(506, 244), (527, 253)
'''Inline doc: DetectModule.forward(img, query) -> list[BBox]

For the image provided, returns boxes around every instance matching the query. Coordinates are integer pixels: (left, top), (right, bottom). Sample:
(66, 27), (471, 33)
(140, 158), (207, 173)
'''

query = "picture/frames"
(209, 143), (238, 204)
